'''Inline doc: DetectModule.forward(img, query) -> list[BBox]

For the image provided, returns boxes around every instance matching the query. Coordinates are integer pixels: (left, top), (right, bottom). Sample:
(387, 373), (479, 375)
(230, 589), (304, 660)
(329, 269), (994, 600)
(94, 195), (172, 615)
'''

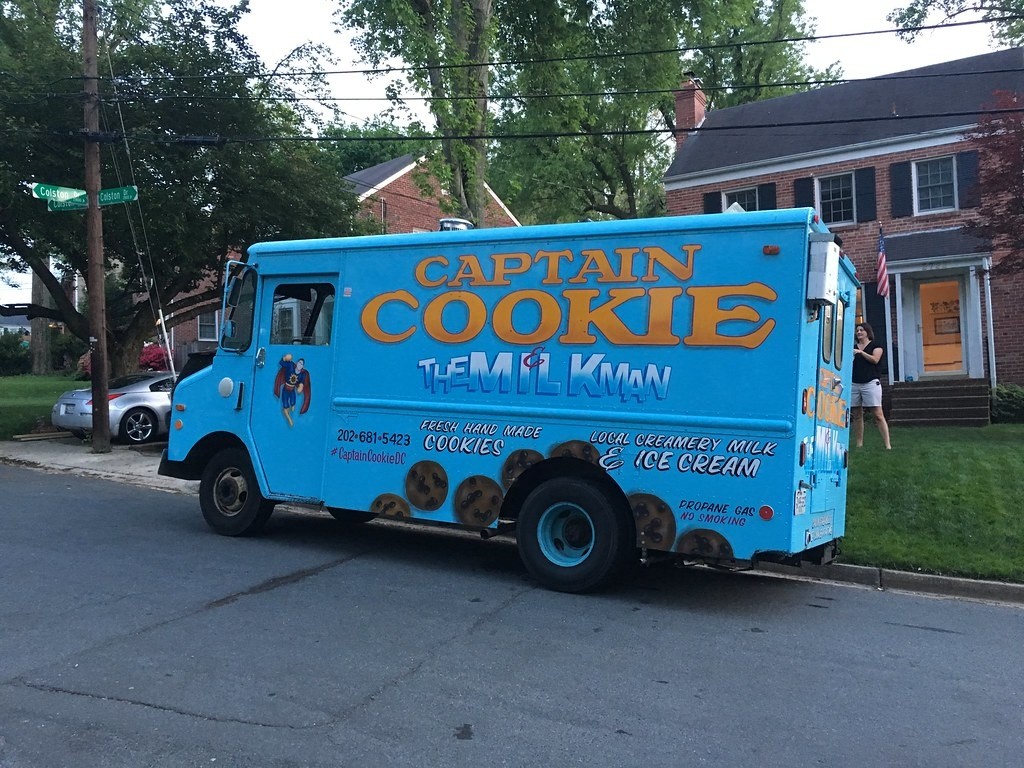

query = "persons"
(849, 323), (894, 450)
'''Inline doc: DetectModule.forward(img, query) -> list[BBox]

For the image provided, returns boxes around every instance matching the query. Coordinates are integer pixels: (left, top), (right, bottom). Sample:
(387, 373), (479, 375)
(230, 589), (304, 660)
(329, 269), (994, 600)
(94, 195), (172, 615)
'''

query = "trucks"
(157, 206), (863, 591)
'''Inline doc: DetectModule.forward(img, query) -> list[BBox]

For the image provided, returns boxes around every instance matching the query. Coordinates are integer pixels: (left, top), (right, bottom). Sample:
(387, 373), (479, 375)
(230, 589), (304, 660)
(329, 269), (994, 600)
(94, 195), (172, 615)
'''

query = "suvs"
(165, 349), (215, 429)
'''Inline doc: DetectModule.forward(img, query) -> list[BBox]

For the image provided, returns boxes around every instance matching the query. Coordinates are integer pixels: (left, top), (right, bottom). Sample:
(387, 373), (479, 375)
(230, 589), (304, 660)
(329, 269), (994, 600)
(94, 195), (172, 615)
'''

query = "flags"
(876, 227), (890, 299)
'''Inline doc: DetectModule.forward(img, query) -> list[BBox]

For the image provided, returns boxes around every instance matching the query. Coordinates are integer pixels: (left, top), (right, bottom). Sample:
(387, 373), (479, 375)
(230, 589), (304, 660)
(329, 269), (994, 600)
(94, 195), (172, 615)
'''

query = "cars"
(51, 371), (178, 443)
